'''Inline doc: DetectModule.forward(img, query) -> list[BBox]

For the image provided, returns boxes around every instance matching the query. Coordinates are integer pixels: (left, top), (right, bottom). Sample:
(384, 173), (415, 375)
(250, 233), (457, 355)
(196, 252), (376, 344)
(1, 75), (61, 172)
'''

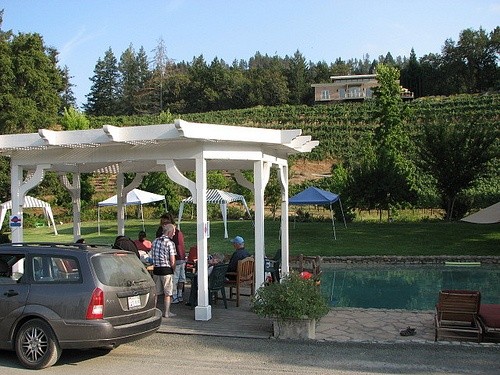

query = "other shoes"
(177, 297), (183, 302)
(166, 312), (176, 318)
(170, 298), (179, 303)
(400, 326), (416, 336)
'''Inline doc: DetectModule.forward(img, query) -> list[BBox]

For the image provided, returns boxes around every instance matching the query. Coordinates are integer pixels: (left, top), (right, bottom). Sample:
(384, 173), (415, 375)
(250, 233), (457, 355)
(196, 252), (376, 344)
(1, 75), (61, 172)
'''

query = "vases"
(269, 310), (317, 340)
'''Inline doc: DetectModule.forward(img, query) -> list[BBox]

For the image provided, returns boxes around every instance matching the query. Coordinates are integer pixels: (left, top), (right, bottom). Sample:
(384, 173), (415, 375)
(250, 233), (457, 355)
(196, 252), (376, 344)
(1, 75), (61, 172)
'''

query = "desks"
(145, 256), (220, 308)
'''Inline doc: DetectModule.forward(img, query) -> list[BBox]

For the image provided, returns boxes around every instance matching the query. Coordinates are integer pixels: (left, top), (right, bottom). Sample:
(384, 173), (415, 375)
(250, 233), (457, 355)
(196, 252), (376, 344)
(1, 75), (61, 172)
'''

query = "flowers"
(246, 271), (330, 323)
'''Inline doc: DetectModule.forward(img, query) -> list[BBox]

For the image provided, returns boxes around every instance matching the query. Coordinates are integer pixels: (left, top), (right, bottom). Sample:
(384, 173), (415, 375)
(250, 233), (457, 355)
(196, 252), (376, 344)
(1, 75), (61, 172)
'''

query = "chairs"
(477, 303), (500, 343)
(214, 255), (255, 307)
(432, 289), (484, 342)
(16, 257), (41, 284)
(190, 262), (231, 310)
(0, 259), (11, 279)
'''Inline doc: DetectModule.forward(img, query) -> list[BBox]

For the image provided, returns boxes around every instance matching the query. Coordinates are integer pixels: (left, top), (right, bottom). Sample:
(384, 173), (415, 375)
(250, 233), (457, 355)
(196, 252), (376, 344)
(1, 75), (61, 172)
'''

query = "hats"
(229, 236), (244, 243)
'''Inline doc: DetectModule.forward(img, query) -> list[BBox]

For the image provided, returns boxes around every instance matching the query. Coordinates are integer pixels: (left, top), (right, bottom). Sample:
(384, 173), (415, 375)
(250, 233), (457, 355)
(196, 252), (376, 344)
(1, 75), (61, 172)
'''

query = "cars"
(0, 241), (162, 370)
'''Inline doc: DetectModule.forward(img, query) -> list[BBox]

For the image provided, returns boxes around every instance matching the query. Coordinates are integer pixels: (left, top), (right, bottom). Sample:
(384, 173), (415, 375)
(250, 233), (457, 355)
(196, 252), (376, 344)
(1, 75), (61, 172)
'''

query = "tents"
(98, 188), (168, 235)
(281, 186), (347, 240)
(174, 188), (255, 238)
(0, 195), (59, 236)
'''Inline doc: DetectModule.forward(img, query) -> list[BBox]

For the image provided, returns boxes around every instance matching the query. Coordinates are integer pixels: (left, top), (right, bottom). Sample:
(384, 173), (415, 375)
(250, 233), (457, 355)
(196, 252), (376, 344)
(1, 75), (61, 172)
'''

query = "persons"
(134, 231), (151, 252)
(77, 239), (86, 245)
(0, 230), (12, 243)
(188, 243), (197, 268)
(160, 213), (186, 303)
(185, 251), (225, 305)
(149, 224), (178, 317)
(115, 235), (141, 259)
(223, 236), (251, 284)
